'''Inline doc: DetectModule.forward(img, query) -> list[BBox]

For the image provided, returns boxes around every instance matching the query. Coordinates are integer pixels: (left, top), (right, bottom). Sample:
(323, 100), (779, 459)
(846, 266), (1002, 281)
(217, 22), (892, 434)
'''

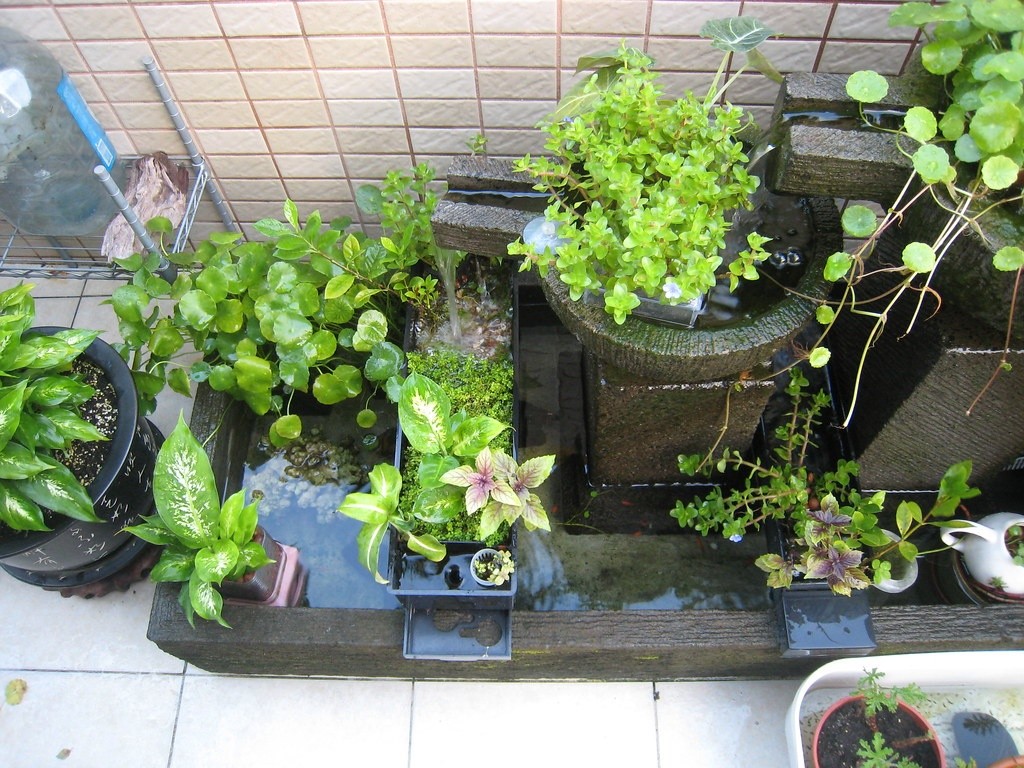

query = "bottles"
(0, 26), (126, 237)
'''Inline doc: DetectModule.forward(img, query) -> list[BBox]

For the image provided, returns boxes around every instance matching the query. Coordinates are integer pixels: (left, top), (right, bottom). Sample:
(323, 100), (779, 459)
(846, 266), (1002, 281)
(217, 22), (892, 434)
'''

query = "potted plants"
(0, 0), (1024, 768)
